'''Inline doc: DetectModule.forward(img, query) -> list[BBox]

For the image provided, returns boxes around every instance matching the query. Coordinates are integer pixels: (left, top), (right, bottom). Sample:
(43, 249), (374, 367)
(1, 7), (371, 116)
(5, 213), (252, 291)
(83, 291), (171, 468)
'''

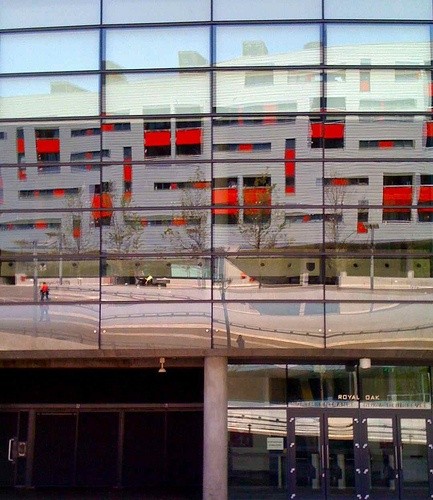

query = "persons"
(379, 458), (394, 488)
(40, 282), (49, 301)
(145, 272), (154, 288)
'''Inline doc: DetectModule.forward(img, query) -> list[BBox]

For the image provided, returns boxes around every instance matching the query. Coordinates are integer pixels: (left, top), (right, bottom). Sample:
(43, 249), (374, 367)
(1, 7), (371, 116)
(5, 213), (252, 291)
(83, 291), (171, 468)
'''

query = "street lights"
(363, 222), (380, 292)
(187, 226), (209, 289)
(47, 232), (64, 286)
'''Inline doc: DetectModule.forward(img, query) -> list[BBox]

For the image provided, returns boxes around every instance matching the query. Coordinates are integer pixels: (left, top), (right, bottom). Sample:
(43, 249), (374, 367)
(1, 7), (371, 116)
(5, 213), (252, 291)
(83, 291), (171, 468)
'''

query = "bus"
(227, 402), (432, 499)
